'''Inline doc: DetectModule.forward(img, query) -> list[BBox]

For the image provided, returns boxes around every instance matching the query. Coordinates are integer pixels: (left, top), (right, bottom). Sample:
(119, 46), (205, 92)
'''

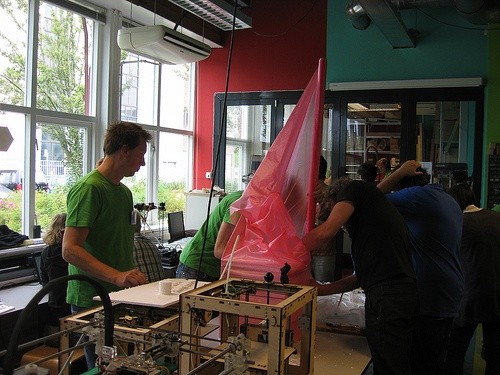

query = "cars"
(0, 184), (21, 210)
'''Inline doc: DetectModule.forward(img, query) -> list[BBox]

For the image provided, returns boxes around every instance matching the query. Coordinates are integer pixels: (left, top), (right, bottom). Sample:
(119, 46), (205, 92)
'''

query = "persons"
(40, 121), (165, 371)
(174, 155), (500, 375)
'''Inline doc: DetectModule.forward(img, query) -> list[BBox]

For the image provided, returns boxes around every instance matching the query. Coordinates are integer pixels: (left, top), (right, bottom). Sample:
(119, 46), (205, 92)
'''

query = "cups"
(159, 280), (171, 294)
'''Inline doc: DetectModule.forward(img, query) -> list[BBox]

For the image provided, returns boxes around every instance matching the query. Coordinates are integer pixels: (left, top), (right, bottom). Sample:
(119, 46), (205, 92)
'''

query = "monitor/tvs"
(168, 210), (186, 243)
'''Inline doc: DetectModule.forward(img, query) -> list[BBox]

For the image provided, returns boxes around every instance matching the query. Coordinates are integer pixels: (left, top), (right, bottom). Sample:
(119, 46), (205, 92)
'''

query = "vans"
(0, 169), (51, 197)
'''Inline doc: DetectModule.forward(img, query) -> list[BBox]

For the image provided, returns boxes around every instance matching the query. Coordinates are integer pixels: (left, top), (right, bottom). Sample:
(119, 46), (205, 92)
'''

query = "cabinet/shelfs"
(363, 118), (402, 183)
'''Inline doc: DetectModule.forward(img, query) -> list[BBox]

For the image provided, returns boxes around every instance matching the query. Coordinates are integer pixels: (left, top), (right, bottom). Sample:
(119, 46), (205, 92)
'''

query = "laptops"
(33, 252), (42, 285)
